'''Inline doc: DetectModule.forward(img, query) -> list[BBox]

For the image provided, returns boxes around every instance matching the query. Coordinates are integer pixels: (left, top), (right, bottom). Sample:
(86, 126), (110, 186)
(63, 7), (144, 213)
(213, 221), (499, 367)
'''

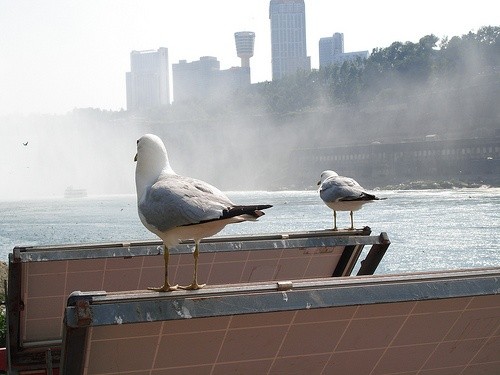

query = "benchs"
(0, 225), (392, 375)
(60, 266), (500, 375)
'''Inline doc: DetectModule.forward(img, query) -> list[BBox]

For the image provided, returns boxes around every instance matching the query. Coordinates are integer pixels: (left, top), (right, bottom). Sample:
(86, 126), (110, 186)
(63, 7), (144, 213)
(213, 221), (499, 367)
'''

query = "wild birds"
(317, 169), (388, 231)
(134, 133), (274, 292)
